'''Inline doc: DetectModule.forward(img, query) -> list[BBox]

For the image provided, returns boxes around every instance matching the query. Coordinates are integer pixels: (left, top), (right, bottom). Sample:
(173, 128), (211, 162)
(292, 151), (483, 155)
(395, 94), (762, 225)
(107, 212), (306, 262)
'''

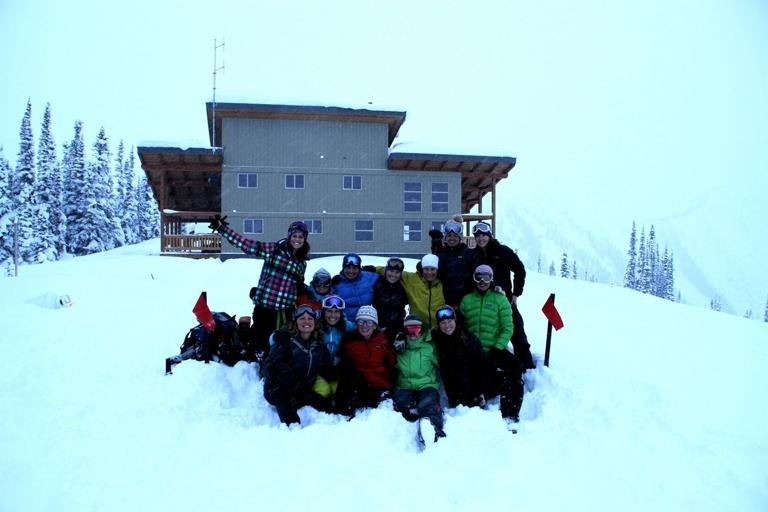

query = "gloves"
(208, 214), (230, 233)
(485, 346), (500, 370)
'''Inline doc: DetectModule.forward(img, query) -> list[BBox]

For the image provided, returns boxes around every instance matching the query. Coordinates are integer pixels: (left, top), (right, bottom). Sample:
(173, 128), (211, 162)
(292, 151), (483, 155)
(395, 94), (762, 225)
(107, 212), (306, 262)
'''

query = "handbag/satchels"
(180, 312), (258, 366)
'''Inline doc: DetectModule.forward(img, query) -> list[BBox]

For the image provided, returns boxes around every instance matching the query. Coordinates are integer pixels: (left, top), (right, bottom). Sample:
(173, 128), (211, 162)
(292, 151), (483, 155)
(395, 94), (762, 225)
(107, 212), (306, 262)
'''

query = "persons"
(429, 215), (474, 306)
(165, 310), (238, 372)
(468, 222), (536, 373)
(206, 212), (311, 361)
(260, 252), (525, 442)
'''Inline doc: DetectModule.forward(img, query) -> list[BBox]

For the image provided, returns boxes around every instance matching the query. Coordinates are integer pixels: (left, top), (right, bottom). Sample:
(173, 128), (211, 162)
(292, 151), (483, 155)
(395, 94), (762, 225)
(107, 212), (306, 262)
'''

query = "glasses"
(472, 271), (493, 283)
(472, 223), (491, 234)
(406, 325), (421, 335)
(322, 295), (346, 310)
(356, 319), (374, 327)
(288, 224), (305, 232)
(436, 307), (454, 321)
(343, 255), (360, 267)
(294, 305), (316, 317)
(389, 259), (404, 270)
(445, 223), (463, 234)
(314, 277), (332, 286)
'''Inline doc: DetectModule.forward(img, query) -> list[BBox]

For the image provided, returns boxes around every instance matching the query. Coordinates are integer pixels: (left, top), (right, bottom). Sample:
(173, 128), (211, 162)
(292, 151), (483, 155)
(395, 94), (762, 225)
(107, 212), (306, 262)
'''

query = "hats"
(355, 305), (379, 324)
(288, 221), (309, 239)
(473, 264), (494, 282)
(403, 315), (424, 326)
(313, 268), (332, 288)
(421, 254), (439, 269)
(444, 215), (463, 240)
(435, 305), (457, 322)
